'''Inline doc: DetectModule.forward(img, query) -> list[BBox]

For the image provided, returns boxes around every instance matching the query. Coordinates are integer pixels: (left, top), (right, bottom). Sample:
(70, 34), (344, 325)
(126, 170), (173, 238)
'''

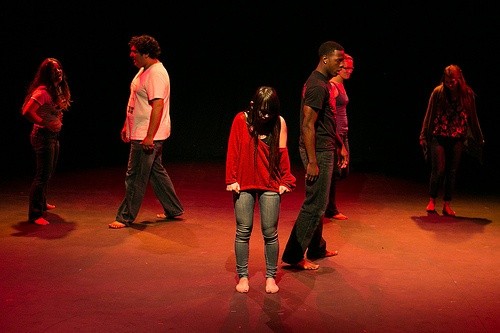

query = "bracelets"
(420, 136), (426, 139)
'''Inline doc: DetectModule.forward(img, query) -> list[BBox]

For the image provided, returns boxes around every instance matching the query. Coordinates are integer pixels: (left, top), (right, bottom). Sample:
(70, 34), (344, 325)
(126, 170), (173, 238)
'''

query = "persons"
(325, 54), (354, 221)
(22, 58), (72, 225)
(226, 86), (296, 294)
(420, 66), (484, 216)
(281, 41), (349, 270)
(109, 36), (185, 229)
